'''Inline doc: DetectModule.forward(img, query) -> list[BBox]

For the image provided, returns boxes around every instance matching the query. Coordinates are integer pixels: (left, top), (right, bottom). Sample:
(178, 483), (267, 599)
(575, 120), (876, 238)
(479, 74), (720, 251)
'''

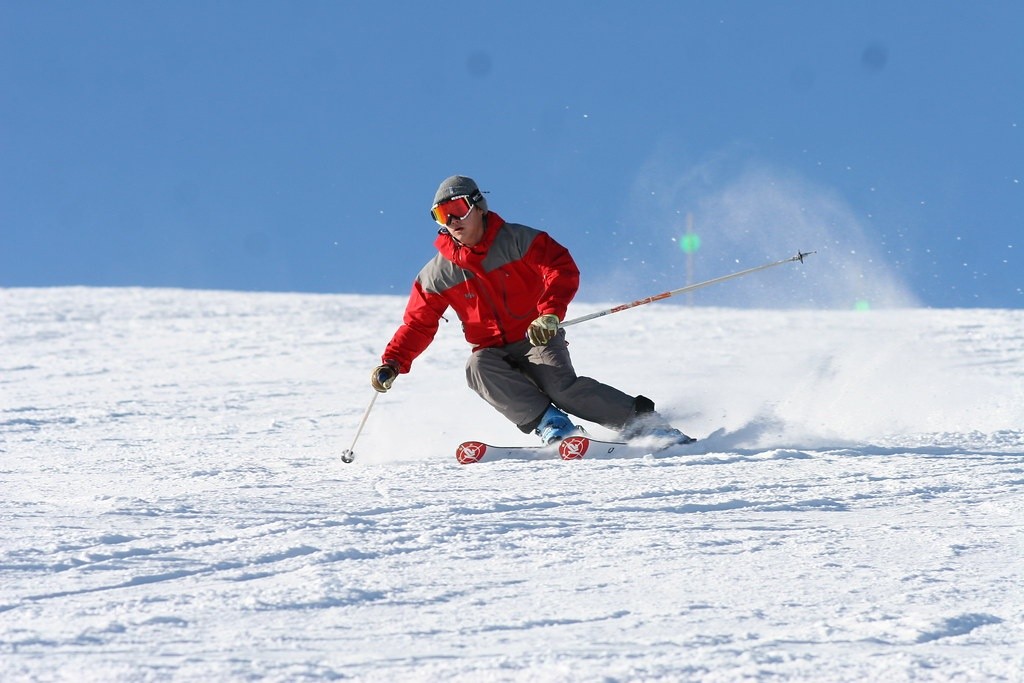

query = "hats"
(433, 175), (488, 216)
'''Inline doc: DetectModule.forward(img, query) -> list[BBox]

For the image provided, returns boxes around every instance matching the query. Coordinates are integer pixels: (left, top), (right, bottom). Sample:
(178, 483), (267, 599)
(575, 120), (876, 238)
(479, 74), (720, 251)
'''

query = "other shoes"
(654, 429), (690, 444)
(535, 404), (574, 446)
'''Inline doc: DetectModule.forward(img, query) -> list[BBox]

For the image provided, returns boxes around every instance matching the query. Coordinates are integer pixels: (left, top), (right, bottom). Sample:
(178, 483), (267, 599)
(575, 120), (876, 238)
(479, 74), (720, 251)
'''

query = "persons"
(372, 176), (691, 447)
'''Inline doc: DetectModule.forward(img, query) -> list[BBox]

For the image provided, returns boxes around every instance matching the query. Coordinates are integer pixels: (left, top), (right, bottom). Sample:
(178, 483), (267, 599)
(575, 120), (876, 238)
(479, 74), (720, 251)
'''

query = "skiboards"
(455, 436), (663, 466)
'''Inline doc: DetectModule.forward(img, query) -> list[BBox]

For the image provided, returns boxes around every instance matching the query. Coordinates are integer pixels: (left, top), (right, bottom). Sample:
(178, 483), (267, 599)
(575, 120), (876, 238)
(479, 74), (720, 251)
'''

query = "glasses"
(430, 196), (472, 226)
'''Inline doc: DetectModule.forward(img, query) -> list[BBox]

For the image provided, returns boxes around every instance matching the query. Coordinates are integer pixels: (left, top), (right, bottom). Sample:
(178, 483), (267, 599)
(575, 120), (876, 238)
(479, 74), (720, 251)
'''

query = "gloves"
(527, 314), (559, 347)
(371, 364), (396, 393)
